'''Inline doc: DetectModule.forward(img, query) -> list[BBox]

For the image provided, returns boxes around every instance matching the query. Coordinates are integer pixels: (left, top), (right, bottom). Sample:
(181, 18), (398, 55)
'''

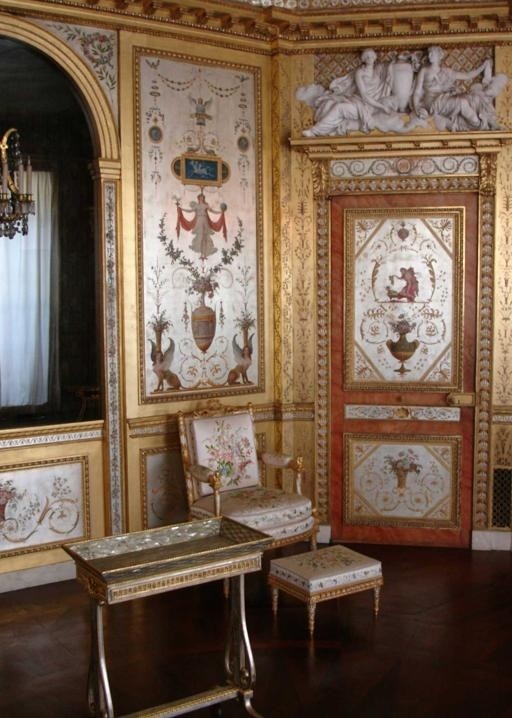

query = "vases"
(0, 504), (8, 522)
(386, 335), (420, 375)
(191, 307), (216, 354)
(391, 61), (414, 112)
(397, 472), (406, 489)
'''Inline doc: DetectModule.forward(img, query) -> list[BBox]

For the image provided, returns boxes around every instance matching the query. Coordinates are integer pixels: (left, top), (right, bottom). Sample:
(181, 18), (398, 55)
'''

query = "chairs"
(178, 400), (320, 601)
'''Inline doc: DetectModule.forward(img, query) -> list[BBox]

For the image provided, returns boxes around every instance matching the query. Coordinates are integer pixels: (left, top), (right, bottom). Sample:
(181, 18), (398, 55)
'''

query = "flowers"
(0, 477), (80, 542)
(204, 421), (255, 485)
(158, 211), (244, 304)
(385, 449), (422, 475)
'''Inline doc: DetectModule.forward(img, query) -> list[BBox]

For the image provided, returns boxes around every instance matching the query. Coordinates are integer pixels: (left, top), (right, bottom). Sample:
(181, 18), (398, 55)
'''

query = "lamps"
(0, 127), (35, 240)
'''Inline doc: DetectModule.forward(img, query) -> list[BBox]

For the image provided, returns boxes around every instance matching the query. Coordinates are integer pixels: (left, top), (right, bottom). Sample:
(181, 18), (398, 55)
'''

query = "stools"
(269, 544), (383, 638)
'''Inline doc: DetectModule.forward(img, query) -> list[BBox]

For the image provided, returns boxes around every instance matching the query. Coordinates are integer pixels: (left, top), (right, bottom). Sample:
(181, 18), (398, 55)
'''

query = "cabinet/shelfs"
(61, 516), (274, 718)
